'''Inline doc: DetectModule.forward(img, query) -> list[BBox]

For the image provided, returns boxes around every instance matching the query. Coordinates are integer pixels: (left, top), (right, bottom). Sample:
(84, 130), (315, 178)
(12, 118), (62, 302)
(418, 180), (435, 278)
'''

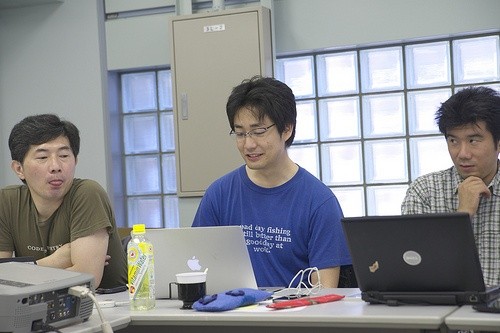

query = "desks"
(446, 303), (500, 333)
(92, 287), (462, 333)
(43, 308), (132, 333)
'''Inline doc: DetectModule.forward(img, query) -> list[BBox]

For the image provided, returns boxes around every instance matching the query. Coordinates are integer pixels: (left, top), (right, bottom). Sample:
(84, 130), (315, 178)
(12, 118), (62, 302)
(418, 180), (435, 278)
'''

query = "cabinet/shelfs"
(167, 5), (275, 197)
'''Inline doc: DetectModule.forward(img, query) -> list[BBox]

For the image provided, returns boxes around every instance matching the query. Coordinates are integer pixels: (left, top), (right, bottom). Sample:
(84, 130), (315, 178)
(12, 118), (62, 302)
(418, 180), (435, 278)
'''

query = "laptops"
(340, 212), (500, 306)
(128, 226), (285, 300)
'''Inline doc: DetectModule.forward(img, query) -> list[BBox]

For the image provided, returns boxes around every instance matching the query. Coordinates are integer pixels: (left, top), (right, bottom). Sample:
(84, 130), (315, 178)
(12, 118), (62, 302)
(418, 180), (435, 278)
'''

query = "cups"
(169, 273), (206, 307)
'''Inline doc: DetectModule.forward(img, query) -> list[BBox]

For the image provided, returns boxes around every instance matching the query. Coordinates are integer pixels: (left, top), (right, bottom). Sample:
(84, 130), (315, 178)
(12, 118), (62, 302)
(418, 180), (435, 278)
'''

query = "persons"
(0, 114), (130, 294)
(191, 75), (353, 288)
(401, 87), (500, 289)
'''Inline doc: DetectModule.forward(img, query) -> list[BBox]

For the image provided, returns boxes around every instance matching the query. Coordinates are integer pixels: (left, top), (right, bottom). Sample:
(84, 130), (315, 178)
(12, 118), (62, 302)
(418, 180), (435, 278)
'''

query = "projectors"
(0, 260), (95, 333)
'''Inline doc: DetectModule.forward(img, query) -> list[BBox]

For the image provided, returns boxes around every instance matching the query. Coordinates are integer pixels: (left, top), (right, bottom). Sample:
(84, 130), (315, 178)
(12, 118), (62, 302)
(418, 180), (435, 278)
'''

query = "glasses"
(229, 124), (277, 141)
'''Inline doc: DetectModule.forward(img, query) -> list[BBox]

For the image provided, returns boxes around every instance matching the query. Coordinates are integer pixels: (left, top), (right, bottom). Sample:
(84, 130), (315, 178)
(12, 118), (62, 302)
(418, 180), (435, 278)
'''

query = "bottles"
(127, 224), (155, 310)
